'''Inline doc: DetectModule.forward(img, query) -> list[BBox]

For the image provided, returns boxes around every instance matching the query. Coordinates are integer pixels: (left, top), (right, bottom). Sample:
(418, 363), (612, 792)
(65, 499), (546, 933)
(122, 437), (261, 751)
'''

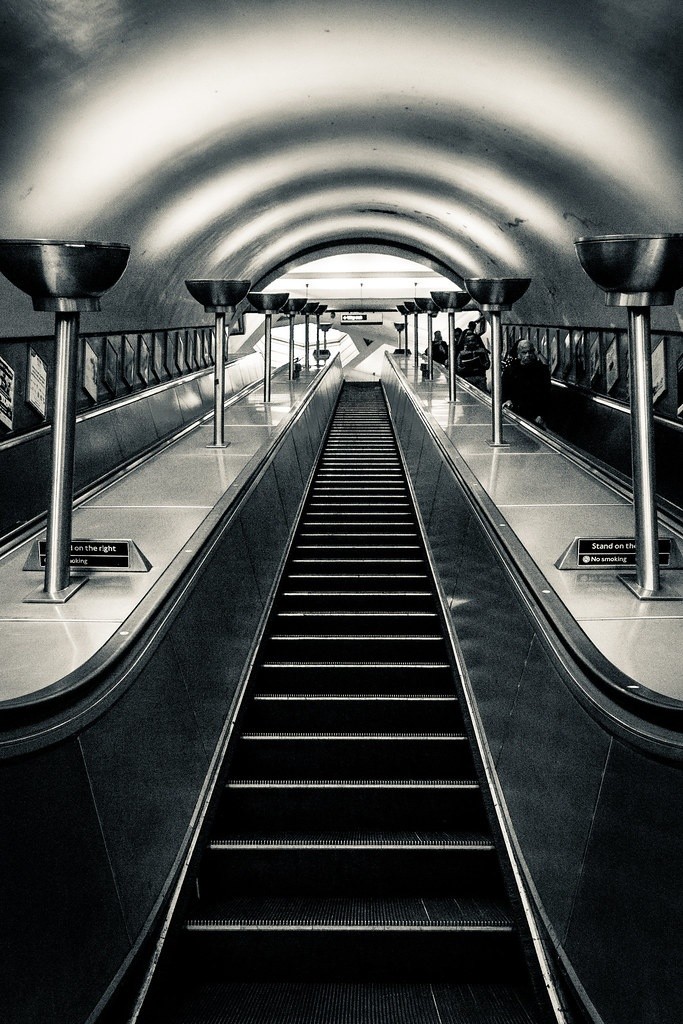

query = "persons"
(501, 338), (552, 426)
(429, 317), (490, 389)
